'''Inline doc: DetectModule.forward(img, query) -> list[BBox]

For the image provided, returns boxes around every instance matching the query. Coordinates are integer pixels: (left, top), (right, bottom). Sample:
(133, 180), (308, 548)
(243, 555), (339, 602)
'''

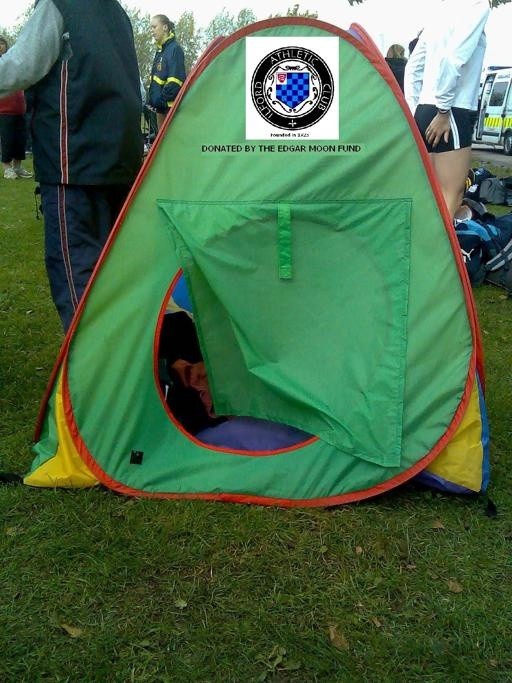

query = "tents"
(20, 14), (492, 509)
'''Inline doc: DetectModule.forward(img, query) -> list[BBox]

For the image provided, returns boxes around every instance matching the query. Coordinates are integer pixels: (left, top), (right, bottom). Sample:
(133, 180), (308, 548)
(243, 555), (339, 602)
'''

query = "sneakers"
(12, 165), (33, 178)
(4, 167), (18, 180)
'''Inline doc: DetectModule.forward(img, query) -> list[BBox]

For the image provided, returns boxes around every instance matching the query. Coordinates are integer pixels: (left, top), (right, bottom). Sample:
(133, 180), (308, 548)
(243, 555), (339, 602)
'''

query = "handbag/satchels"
(454, 167), (511, 295)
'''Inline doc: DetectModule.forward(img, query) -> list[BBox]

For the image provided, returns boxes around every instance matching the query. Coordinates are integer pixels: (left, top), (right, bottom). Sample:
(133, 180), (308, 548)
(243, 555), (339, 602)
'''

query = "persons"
(384, 0), (493, 225)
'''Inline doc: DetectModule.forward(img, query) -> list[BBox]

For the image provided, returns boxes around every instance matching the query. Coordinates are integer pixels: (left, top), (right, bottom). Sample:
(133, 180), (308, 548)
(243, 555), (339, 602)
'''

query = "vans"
(470, 67), (512, 155)
